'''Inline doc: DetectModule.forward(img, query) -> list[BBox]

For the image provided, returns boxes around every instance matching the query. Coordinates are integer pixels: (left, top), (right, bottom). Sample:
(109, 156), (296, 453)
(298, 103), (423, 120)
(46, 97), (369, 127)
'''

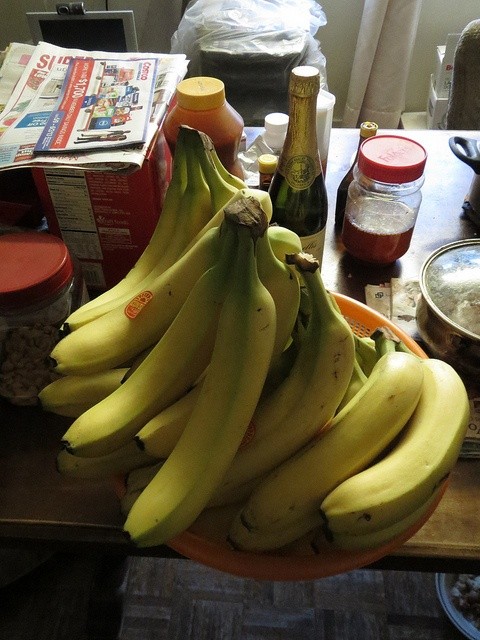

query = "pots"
(414, 240), (479, 367)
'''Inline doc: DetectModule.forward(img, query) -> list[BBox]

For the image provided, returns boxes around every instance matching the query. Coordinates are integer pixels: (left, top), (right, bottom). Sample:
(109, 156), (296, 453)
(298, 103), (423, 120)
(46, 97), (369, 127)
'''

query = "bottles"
(257, 154), (278, 190)
(334, 121), (379, 230)
(264, 112), (290, 149)
(267, 65), (328, 276)
(163, 76), (244, 185)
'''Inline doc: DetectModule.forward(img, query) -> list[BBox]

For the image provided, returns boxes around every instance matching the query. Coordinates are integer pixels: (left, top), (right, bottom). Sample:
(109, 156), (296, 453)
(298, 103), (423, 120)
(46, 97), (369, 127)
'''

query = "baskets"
(115, 291), (451, 581)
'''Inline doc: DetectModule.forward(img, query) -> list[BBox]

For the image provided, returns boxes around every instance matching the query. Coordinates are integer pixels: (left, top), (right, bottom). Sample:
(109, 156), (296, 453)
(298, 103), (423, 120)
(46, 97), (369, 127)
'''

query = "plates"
(434, 572), (480, 638)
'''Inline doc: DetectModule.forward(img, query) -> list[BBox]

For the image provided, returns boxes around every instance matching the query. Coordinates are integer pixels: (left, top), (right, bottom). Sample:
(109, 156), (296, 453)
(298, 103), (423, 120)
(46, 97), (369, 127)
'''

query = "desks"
(1, 127), (480, 580)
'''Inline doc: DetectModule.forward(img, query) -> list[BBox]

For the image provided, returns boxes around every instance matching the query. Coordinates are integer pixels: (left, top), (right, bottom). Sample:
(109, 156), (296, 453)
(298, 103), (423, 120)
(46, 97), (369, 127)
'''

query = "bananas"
(57, 125), (246, 335)
(52, 199), (299, 543)
(120, 252), (354, 514)
(233, 326), (470, 549)
